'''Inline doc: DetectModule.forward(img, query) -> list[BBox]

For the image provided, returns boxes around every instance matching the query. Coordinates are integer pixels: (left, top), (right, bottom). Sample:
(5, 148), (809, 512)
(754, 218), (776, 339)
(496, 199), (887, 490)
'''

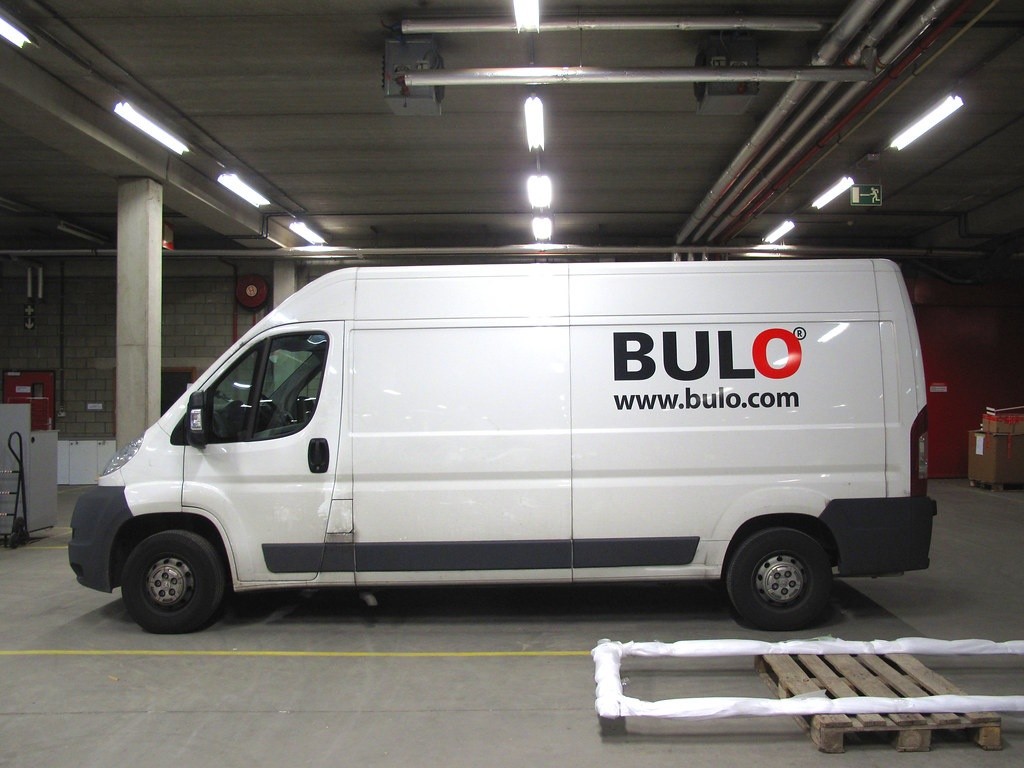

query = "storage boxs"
(964, 430), (1024, 494)
(982, 413), (1024, 435)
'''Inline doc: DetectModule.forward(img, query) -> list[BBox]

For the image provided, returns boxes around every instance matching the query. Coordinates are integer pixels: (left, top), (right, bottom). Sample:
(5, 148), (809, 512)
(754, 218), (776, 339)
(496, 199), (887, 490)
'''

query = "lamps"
(1, 1), (972, 264)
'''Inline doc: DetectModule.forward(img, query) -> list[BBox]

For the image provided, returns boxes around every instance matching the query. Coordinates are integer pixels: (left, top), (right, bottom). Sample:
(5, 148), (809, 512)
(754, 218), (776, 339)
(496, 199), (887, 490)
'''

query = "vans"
(66, 256), (939, 638)
